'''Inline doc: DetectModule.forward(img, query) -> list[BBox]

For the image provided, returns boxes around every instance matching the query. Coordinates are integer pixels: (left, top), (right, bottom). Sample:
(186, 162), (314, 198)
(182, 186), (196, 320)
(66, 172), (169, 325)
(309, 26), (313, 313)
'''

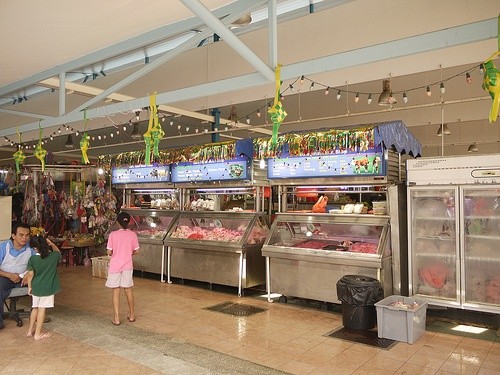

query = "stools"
(3, 287), (39, 326)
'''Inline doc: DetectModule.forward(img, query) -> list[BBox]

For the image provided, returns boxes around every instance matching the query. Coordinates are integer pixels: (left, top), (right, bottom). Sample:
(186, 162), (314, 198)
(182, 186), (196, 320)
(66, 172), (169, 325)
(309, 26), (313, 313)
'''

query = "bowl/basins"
(372, 201), (386, 215)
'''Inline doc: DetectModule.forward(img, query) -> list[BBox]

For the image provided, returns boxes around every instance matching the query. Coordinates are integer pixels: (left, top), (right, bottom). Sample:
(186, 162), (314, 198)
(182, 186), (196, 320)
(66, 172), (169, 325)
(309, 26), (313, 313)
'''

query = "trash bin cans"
(337, 275), (384, 330)
(375, 294), (429, 344)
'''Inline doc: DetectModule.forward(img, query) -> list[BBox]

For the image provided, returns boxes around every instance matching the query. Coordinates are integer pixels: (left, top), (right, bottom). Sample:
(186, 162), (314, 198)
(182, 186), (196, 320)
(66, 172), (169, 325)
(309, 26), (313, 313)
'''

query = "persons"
(104, 212), (140, 325)
(0, 223), (51, 329)
(26, 234), (62, 339)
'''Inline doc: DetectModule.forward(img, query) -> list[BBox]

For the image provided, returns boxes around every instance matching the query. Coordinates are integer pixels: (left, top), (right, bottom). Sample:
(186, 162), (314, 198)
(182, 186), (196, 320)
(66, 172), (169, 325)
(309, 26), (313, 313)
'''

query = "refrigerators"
(405, 156), (500, 316)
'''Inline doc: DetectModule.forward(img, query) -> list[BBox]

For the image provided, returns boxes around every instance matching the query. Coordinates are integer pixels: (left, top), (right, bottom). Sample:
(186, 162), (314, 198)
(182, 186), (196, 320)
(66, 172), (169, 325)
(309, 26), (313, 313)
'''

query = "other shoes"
(43, 318), (51, 323)
(0, 323), (4, 329)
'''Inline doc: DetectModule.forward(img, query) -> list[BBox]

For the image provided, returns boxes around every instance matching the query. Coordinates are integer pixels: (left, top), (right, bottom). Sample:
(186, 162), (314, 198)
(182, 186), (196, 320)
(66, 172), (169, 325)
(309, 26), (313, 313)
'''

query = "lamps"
(437, 124), (451, 137)
(378, 80), (397, 106)
(467, 144), (478, 152)
(65, 135), (74, 148)
(221, 11), (251, 28)
(225, 106), (242, 128)
(131, 123), (142, 137)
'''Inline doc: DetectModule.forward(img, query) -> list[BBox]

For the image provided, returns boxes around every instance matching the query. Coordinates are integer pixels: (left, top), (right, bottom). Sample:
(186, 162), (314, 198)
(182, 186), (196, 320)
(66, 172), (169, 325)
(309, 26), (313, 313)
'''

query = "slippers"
(26, 330), (35, 337)
(127, 314), (135, 322)
(34, 332), (53, 340)
(112, 319), (120, 325)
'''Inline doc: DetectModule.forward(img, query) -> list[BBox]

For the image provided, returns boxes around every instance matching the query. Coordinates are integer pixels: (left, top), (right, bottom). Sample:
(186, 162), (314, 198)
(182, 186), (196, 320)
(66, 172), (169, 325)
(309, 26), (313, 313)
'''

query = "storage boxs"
(91, 256), (110, 279)
(374, 295), (430, 344)
